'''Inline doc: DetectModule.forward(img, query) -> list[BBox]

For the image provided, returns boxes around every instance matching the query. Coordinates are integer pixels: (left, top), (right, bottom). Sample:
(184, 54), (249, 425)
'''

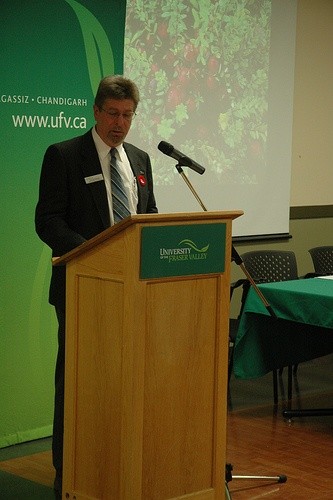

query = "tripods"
(176, 166), (289, 500)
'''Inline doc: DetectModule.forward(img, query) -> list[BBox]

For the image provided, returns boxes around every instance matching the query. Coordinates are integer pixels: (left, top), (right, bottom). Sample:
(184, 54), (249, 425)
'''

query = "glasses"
(99, 106), (136, 121)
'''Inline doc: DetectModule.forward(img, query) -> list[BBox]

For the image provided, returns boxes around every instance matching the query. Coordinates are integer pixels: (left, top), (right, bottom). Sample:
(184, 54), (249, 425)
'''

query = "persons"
(34, 75), (158, 500)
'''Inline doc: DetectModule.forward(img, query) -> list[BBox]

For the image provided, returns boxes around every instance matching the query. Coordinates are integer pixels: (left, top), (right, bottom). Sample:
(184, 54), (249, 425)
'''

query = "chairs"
(240, 246), (333, 403)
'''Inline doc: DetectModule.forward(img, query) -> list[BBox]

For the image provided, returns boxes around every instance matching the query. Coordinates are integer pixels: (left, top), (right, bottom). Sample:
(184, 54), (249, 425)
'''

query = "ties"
(109, 148), (131, 224)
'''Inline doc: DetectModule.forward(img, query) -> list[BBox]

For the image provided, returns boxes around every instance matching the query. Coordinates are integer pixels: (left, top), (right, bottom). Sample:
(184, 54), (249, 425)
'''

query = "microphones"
(158, 141), (206, 175)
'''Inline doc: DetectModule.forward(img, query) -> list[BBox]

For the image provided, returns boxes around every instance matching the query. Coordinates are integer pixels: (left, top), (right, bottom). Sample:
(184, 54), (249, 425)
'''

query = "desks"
(233, 275), (333, 423)
